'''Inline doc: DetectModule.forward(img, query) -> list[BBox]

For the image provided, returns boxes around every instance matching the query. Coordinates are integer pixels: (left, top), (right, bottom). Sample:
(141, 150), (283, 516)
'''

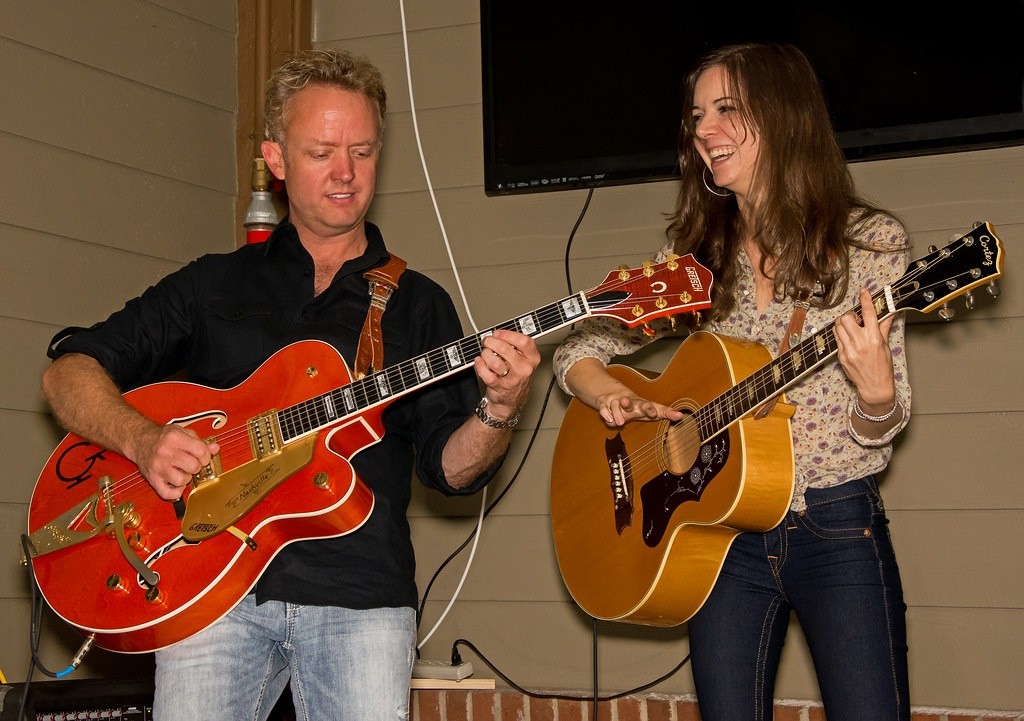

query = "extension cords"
(413, 658), (473, 681)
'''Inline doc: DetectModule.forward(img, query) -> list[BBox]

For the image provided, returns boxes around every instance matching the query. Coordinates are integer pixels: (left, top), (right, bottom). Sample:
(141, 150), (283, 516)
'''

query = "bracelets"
(475, 395), (523, 428)
(853, 396), (897, 422)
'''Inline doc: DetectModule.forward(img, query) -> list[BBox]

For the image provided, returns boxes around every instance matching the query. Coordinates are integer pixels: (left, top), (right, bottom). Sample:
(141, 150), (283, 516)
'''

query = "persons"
(553, 44), (910, 721)
(42, 48), (542, 721)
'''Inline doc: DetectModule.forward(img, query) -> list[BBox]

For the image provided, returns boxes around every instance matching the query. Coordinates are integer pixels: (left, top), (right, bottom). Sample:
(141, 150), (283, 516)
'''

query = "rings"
(500, 362), (510, 377)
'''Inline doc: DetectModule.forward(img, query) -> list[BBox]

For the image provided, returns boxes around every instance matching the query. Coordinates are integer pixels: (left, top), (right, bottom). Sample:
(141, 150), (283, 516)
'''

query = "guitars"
(550, 219), (1004, 631)
(27, 250), (715, 655)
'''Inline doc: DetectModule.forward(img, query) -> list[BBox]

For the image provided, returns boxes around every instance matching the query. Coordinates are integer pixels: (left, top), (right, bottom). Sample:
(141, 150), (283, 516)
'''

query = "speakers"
(0, 677), (153, 721)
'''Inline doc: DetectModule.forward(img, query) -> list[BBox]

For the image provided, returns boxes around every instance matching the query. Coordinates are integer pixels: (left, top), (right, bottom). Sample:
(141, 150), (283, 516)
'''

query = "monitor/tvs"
(480, 0), (1024, 197)
